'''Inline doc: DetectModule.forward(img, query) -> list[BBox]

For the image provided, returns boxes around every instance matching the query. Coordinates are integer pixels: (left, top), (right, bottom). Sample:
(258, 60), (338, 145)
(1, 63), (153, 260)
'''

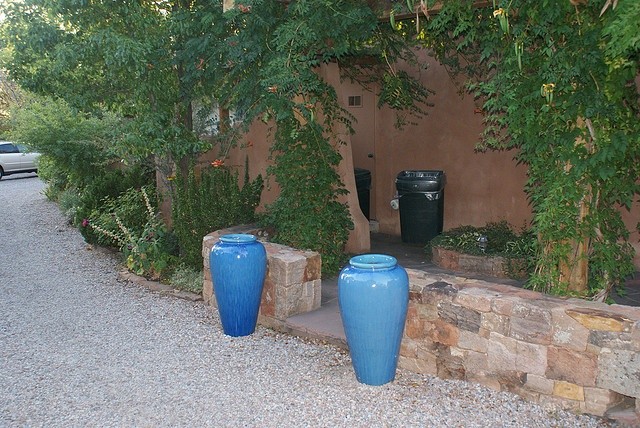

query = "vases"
(337, 254), (409, 386)
(208, 232), (267, 337)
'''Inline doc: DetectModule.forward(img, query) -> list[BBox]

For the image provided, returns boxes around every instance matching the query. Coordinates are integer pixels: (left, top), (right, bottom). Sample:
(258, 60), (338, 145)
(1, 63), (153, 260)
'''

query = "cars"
(0, 142), (42, 179)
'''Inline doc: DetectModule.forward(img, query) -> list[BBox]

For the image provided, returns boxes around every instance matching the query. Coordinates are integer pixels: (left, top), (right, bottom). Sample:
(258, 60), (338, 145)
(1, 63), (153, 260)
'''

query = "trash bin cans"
(395, 170), (444, 243)
(354, 167), (371, 221)
